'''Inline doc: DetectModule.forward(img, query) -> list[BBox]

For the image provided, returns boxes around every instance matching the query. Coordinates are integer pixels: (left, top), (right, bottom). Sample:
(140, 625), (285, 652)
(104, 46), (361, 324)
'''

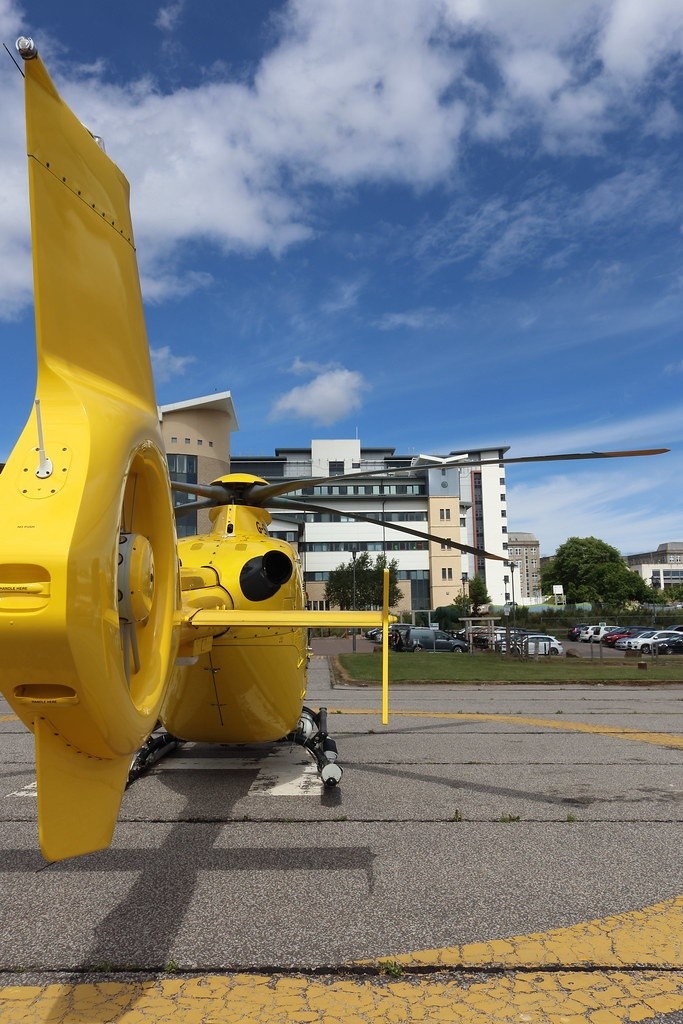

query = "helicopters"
(1, 32), (673, 864)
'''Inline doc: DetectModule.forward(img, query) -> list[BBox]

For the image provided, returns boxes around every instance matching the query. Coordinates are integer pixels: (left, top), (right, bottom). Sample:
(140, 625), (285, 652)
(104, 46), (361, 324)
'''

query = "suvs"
(403, 628), (469, 653)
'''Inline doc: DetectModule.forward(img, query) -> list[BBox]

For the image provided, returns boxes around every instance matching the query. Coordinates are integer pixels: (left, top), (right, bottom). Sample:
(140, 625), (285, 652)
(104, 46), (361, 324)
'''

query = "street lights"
(348, 548), (361, 653)
(509, 561), (516, 627)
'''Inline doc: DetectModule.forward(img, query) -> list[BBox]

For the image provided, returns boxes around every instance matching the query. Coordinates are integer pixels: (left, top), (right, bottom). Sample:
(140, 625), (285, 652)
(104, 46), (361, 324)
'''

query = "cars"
(510, 634), (564, 655)
(615, 631), (649, 651)
(593, 626), (624, 643)
(580, 625), (601, 643)
(365, 622), (430, 649)
(568, 623), (590, 642)
(628, 631), (683, 654)
(456, 626), (549, 654)
(665, 625), (683, 632)
(652, 635), (682, 652)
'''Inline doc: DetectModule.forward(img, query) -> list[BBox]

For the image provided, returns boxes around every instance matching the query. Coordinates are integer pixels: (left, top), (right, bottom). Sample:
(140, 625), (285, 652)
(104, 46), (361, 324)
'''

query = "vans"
(600, 626), (656, 648)
